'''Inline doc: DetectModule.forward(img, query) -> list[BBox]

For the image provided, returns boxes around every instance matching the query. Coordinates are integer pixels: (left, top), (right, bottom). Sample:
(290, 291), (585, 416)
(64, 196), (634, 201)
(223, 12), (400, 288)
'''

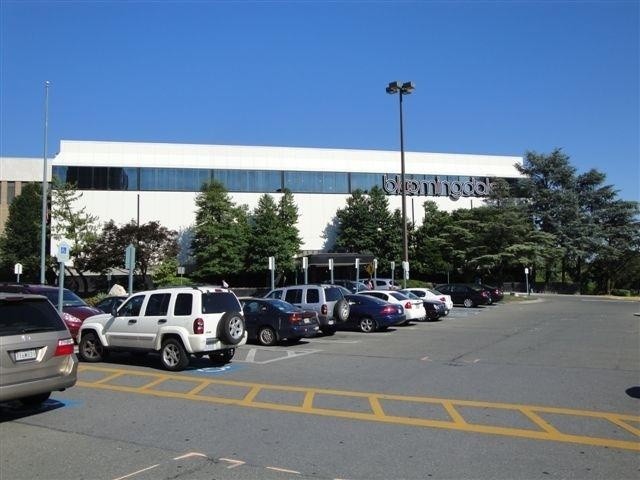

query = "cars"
(355, 291), (427, 321)
(325, 280), (368, 293)
(478, 284), (503, 301)
(1, 285), (105, 340)
(357, 279), (401, 290)
(345, 294), (406, 332)
(0, 293), (78, 408)
(96, 295), (128, 313)
(432, 284), (491, 308)
(398, 291), (444, 321)
(401, 288), (453, 316)
(239, 298), (317, 345)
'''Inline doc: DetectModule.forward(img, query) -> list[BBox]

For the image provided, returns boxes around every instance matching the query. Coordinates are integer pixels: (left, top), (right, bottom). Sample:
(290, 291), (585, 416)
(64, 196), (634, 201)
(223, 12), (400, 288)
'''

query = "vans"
(77, 286), (246, 370)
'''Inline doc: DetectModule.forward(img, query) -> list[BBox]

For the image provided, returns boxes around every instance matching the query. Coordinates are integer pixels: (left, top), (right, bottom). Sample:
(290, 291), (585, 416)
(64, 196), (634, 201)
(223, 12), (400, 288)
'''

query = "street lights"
(387, 80), (415, 284)
(40, 78), (53, 284)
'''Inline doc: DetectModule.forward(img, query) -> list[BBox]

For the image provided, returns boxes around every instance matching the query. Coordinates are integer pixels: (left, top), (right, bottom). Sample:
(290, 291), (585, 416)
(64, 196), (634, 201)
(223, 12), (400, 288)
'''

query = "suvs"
(263, 284), (350, 335)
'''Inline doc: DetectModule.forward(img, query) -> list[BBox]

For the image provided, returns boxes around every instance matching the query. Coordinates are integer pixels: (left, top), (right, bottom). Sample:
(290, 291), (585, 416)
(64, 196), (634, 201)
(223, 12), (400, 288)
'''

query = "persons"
(222, 280), (229, 288)
(367, 278), (372, 290)
